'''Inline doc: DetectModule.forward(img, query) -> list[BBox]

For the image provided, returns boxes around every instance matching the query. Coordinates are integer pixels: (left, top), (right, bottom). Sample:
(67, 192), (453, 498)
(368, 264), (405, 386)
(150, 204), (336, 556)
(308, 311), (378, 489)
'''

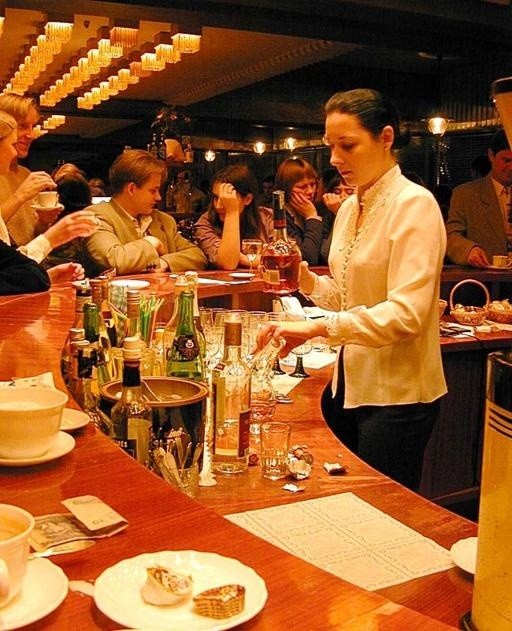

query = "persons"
(446, 129), (512, 270)
(251, 87), (448, 492)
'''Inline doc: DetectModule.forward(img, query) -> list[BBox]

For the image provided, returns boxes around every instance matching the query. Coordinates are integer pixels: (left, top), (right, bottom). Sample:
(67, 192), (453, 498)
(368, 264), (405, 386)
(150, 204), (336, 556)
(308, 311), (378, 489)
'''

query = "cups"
(246, 310), (327, 437)
(149, 463), (200, 503)
(0, 387), (70, 458)
(0, 503), (35, 608)
(439, 298), (448, 319)
(33, 191), (58, 208)
(492, 256), (511, 267)
(260, 421), (290, 481)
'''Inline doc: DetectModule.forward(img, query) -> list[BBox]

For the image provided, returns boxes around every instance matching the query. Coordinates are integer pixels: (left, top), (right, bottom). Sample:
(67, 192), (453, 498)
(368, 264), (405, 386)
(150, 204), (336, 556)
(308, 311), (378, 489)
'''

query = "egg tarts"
(192, 583), (246, 618)
(142, 564), (193, 609)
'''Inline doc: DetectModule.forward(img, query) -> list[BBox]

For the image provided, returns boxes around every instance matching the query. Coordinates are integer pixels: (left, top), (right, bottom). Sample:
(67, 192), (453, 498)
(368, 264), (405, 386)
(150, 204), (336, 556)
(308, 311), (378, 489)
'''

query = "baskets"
(482, 303), (512, 323)
(449, 279), (490, 326)
(438, 299), (448, 319)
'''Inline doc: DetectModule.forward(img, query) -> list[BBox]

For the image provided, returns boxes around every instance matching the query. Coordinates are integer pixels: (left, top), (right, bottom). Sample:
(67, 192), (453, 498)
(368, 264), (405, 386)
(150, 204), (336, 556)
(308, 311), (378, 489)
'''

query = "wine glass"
(242, 238), (262, 280)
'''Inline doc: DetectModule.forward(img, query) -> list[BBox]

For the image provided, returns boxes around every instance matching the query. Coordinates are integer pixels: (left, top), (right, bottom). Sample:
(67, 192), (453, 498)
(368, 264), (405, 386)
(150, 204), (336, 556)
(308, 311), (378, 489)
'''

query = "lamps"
(0, 11), (212, 140)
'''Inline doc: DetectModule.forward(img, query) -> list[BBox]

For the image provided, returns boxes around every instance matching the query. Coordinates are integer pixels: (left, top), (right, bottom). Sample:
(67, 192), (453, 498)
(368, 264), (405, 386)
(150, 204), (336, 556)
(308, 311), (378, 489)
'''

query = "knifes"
(27, 540), (97, 563)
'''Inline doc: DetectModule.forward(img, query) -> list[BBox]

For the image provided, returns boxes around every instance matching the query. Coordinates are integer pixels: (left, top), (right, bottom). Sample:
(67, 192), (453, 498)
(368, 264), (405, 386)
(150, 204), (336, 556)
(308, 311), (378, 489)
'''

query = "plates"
(1, 429), (74, 469)
(30, 204), (64, 211)
(1, 558), (70, 631)
(62, 402), (92, 429)
(228, 271), (255, 280)
(1, 455), (75, 496)
(486, 266), (511, 271)
(448, 536), (477, 578)
(92, 551), (268, 631)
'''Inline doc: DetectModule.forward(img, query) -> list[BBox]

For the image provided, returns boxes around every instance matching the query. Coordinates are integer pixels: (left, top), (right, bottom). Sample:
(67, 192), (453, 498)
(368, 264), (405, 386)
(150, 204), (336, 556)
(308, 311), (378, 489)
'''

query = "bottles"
(166, 173), (192, 215)
(259, 191), (303, 297)
(62, 269), (250, 474)
(184, 142), (194, 162)
(148, 133), (167, 160)
